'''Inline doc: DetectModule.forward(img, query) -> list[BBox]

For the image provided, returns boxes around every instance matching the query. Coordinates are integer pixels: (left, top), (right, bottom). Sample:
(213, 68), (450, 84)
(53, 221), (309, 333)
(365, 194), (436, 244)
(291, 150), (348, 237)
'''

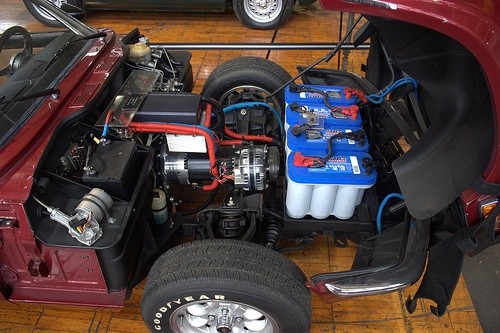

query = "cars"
(24, 0), (316, 29)
(0, 0), (500, 332)
